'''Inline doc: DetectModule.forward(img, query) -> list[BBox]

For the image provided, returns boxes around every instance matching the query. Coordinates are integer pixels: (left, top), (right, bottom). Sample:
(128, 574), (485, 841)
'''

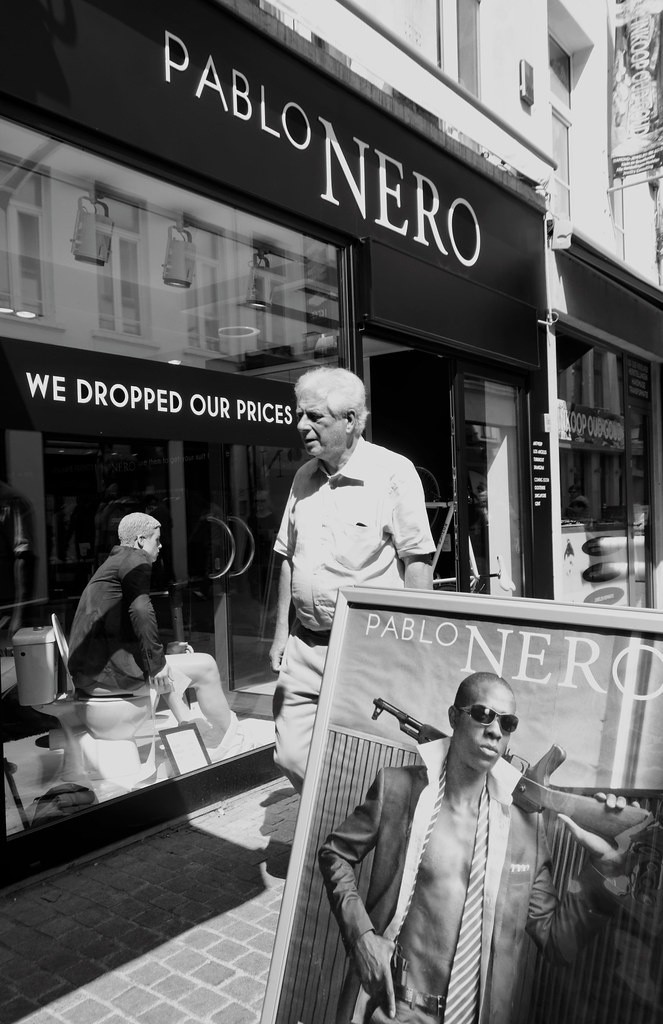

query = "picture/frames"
(258, 583), (663, 1024)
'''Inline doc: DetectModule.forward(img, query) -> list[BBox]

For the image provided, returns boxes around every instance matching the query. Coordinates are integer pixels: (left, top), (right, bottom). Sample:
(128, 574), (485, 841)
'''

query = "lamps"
(246, 248), (274, 310)
(162, 221), (197, 290)
(69, 190), (115, 267)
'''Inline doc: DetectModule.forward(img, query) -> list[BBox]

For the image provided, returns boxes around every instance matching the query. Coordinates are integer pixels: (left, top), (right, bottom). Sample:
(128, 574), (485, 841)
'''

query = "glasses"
(459, 703), (520, 735)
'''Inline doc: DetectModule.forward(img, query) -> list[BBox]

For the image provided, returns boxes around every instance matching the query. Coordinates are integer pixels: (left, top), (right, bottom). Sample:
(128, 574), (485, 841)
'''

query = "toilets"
(12, 612), (159, 781)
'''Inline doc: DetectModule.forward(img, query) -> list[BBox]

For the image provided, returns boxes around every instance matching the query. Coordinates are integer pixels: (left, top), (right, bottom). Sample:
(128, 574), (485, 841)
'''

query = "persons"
(268, 367), (438, 796)
(318, 670), (643, 1024)
(65, 511), (236, 738)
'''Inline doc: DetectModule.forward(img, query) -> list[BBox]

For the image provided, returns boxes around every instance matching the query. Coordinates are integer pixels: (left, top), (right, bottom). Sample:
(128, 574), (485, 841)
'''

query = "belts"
(379, 982), (445, 1012)
(287, 617), (330, 648)
